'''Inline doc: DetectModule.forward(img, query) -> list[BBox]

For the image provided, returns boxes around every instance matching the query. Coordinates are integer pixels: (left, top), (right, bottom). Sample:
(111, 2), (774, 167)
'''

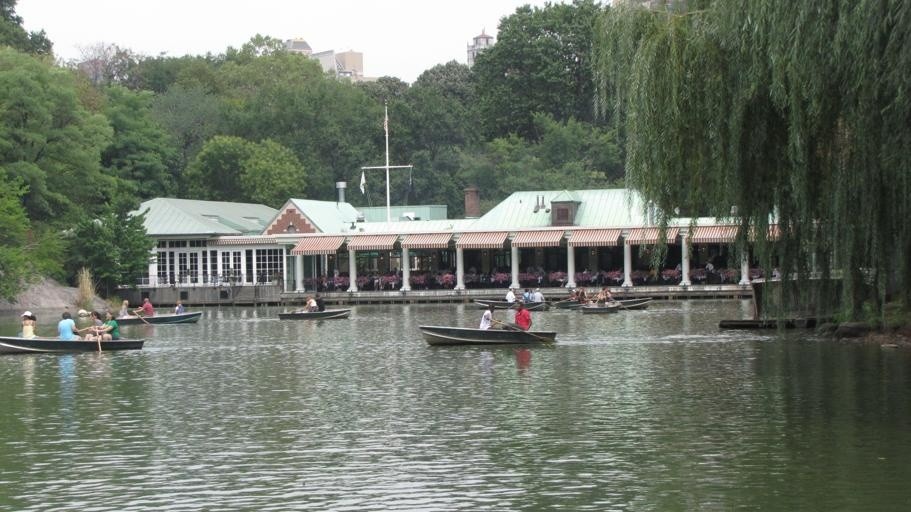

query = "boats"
(0, 336), (144, 353)
(474, 298), (654, 313)
(719, 280), (874, 330)
(419, 325), (557, 346)
(115, 311), (202, 325)
(278, 309), (351, 320)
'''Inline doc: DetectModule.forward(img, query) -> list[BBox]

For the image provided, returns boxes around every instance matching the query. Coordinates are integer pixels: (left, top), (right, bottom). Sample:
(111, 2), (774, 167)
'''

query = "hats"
(21, 311), (32, 318)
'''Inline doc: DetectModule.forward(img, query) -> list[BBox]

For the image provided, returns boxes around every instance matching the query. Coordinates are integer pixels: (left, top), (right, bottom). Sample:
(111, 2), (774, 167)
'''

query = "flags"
(383, 114), (389, 131)
(360, 170), (367, 193)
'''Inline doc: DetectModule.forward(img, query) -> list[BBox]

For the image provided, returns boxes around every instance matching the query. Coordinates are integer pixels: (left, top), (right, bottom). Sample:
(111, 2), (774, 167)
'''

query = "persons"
(58, 312), (82, 340)
(479, 283), (615, 330)
(21, 311), (38, 338)
(84, 313), (102, 340)
(302, 293), (326, 312)
(583, 268), (592, 275)
(92, 312), (120, 341)
(673, 260), (682, 276)
(133, 297), (153, 317)
(705, 260), (715, 272)
(175, 300), (185, 313)
(119, 299), (130, 317)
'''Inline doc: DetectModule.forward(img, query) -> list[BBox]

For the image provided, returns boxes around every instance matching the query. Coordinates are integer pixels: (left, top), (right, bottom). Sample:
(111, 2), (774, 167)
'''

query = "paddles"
(491, 319), (552, 342)
